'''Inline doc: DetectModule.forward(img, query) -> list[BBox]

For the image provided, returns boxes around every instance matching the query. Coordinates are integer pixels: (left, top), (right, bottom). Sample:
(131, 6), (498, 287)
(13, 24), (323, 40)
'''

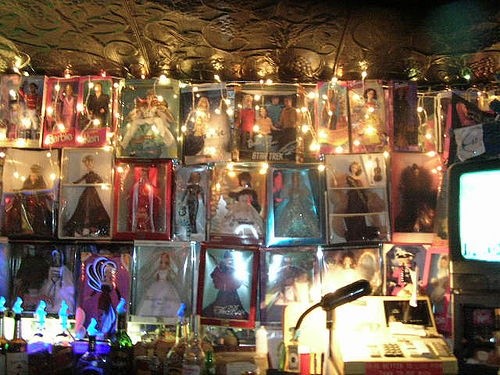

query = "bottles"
(5, 313), (28, 375)
(77, 334), (107, 375)
(204, 349), (218, 375)
(137, 313), (204, 375)
(111, 319), (134, 375)
(0, 312), (9, 375)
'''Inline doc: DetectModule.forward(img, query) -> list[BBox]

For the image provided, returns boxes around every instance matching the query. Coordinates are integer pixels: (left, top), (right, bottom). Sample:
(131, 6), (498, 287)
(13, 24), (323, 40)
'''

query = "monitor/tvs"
(448, 157), (500, 292)
